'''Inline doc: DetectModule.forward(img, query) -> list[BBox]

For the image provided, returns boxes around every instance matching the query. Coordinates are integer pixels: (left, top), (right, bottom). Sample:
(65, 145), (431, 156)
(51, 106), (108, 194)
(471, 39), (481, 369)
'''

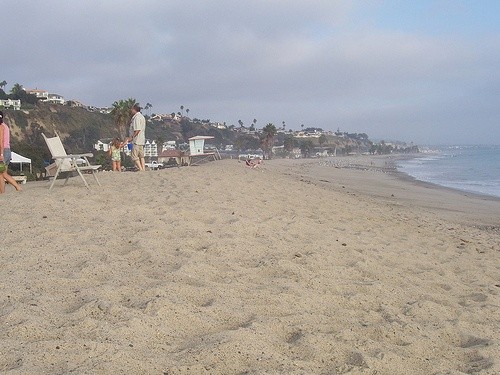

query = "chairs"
(41, 131), (101, 191)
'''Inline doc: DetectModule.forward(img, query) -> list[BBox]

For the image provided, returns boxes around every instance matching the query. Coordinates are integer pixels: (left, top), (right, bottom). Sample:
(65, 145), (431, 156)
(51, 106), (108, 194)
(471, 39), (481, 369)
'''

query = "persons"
(0, 110), (23, 195)
(109, 136), (129, 171)
(127, 104), (147, 170)
(245, 153), (263, 170)
